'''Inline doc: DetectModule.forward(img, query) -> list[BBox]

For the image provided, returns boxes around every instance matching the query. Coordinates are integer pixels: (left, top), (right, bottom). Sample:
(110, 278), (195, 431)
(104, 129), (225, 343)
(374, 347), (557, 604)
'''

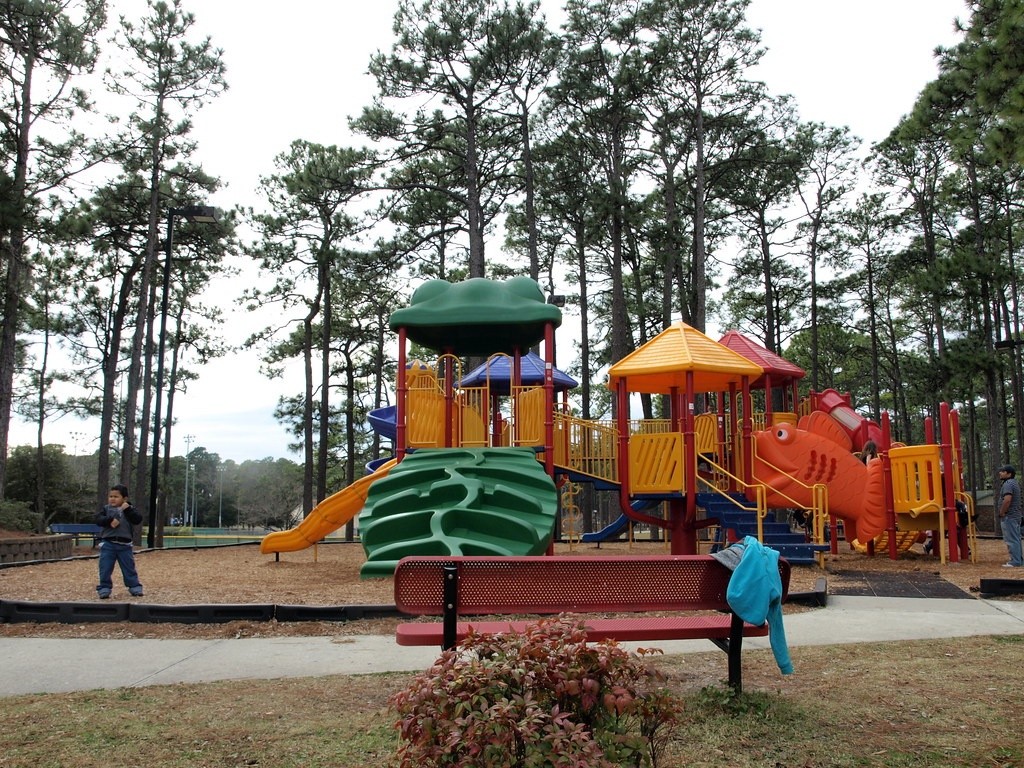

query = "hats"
(998, 465), (1016, 473)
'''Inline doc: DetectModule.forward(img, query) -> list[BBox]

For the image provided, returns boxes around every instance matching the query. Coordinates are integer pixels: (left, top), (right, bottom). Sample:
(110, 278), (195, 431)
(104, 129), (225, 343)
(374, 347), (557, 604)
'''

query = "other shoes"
(1002, 561), (1021, 568)
(923, 545), (930, 555)
(95, 585), (111, 598)
(128, 585), (144, 596)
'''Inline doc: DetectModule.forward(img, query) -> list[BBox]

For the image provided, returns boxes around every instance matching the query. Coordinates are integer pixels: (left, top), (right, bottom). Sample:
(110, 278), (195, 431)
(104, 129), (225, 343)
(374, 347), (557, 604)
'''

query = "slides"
(260, 457), (401, 555)
(819, 388), (904, 463)
(366, 401), (398, 474)
(582, 499), (659, 543)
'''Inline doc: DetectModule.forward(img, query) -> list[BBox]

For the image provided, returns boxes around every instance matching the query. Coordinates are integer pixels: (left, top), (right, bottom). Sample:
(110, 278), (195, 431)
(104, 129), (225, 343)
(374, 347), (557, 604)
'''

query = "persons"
(95, 485), (143, 597)
(698, 452), (719, 474)
(853, 441), (878, 465)
(923, 473), (972, 555)
(792, 509), (830, 543)
(166, 513), (183, 526)
(998, 465), (1024, 567)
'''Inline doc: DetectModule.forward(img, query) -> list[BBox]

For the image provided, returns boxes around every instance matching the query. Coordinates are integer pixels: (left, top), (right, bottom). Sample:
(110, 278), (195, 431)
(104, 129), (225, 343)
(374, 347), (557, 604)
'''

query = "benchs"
(392, 553), (792, 696)
(50, 524), (104, 548)
